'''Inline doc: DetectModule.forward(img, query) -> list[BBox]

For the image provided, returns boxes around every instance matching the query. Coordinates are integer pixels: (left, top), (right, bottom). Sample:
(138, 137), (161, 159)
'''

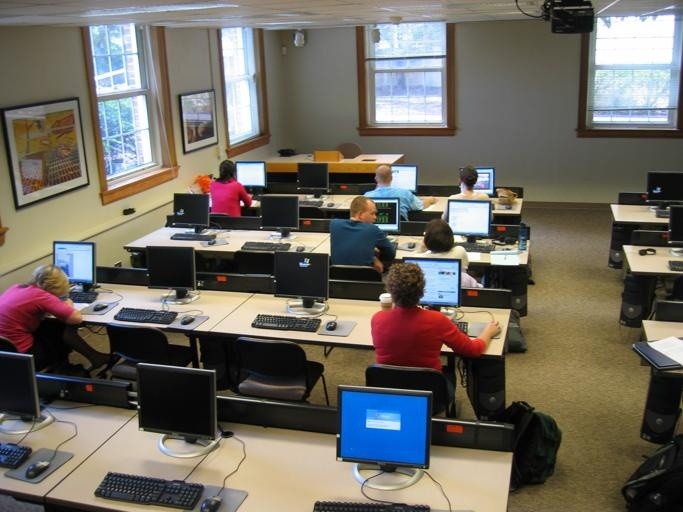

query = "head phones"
(640, 248), (656, 255)
(493, 235), (516, 245)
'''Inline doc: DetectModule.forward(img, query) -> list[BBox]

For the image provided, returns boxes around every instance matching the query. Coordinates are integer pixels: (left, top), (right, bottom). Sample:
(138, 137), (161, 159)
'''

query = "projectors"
(550, 0), (593, 34)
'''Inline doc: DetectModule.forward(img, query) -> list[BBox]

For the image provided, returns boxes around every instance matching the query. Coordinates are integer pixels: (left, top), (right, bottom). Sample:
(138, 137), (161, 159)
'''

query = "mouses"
(180, 316), (195, 325)
(25, 460), (50, 479)
(201, 496), (223, 512)
(506, 205), (512, 209)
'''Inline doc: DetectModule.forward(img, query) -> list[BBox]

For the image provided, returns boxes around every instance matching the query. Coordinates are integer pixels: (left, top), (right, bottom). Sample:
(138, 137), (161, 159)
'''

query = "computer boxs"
(609, 223), (631, 268)
(503, 269), (528, 317)
(640, 377), (681, 444)
(619, 272), (656, 327)
(467, 356), (505, 422)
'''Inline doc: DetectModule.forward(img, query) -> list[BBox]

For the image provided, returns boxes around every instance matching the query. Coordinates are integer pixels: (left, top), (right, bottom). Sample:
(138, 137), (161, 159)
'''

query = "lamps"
(370, 29), (380, 43)
(293, 29), (305, 47)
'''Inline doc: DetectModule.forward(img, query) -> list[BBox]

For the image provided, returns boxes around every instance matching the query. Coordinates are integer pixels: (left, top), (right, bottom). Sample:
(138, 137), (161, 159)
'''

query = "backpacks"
(621, 434), (683, 510)
(505, 400), (562, 489)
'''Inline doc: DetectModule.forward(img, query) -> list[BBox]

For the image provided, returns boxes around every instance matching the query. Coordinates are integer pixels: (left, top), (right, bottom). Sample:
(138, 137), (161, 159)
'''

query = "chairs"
(631, 230), (669, 247)
(644, 298), (683, 322)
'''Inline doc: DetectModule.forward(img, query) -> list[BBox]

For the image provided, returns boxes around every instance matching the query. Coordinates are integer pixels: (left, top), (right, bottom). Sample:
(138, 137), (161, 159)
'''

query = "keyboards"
(0, 442), (32, 469)
(312, 501), (430, 512)
(452, 320), (467, 337)
(251, 314), (321, 332)
(326, 321), (337, 330)
(93, 472), (204, 510)
(656, 211), (670, 218)
(669, 260), (683, 270)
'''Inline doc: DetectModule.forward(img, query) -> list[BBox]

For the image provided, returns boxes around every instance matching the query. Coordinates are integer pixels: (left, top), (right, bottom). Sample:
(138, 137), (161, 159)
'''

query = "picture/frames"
(1, 97), (90, 211)
(178, 89), (219, 155)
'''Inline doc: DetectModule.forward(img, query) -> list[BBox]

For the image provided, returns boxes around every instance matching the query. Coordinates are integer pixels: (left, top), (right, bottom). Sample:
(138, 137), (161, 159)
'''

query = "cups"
(380, 293), (393, 312)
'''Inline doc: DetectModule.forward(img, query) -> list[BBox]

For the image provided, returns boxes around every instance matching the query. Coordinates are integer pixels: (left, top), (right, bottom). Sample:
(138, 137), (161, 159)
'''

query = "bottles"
(518, 222), (528, 250)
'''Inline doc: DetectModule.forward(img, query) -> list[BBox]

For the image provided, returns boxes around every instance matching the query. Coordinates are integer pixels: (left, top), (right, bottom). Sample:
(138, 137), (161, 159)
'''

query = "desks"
(609, 203), (669, 268)
(619, 244), (682, 328)
(639, 319), (683, 446)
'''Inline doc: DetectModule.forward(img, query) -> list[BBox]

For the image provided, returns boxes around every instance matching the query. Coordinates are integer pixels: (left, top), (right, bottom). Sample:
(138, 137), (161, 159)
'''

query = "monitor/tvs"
(668, 205), (683, 244)
(0, 351), (55, 434)
(646, 170), (683, 212)
(135, 362), (222, 458)
(336, 384), (433, 491)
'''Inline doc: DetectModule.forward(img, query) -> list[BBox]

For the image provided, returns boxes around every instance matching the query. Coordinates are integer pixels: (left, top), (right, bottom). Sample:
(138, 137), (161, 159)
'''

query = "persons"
(371, 262), (501, 418)
(210, 159), (252, 217)
(406, 219), (470, 274)
(0, 265), (122, 377)
(441, 166), (494, 223)
(363, 165), (439, 222)
(329, 196), (396, 271)
